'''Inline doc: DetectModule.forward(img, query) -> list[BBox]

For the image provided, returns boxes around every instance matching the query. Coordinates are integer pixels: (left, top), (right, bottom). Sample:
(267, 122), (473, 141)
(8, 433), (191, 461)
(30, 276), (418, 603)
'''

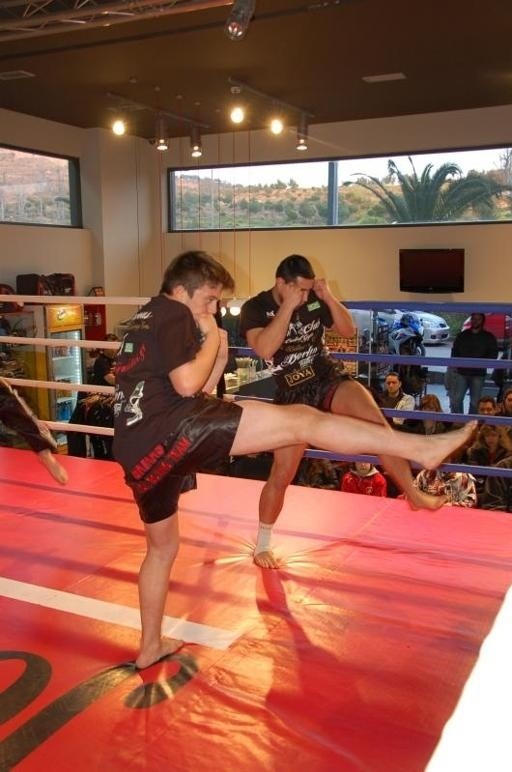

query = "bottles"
(55, 378), (74, 422)
(52, 345), (70, 357)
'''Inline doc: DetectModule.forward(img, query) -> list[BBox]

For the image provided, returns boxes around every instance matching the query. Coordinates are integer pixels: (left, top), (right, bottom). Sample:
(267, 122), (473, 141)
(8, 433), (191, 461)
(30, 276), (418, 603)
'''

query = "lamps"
(152, 115), (170, 151)
(112, 109), (128, 136)
(296, 113), (308, 150)
(188, 126), (202, 158)
(222, 0), (256, 43)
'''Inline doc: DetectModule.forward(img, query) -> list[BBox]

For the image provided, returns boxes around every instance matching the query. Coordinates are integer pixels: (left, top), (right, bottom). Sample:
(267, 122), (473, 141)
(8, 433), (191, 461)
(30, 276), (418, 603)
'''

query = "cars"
(462, 312), (512, 350)
(347, 308), (450, 345)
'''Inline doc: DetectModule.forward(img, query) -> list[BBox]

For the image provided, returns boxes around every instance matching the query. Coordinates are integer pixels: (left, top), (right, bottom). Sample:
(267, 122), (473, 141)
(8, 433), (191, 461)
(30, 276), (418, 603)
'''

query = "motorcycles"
(361, 311), (426, 356)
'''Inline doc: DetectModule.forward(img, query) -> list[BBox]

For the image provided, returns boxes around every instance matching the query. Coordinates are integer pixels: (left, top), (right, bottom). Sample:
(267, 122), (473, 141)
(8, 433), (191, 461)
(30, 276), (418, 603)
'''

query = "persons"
(236, 255), (450, 571)
(112, 249), (480, 671)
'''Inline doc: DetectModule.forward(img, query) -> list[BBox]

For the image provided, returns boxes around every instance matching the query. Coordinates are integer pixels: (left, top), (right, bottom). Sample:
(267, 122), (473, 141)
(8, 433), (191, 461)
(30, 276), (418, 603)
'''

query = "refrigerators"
(9, 303), (89, 455)
